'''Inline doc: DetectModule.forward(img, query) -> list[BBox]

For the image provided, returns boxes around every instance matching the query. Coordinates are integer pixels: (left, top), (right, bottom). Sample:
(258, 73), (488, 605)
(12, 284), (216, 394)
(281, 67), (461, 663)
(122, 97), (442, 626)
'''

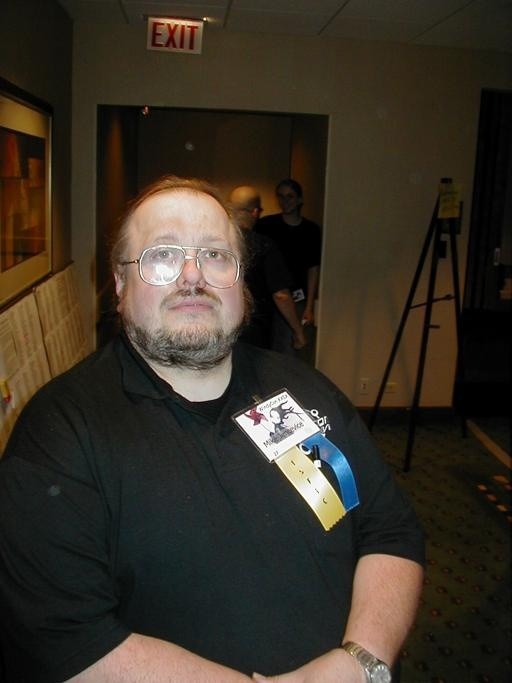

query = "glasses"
(120, 243), (242, 285)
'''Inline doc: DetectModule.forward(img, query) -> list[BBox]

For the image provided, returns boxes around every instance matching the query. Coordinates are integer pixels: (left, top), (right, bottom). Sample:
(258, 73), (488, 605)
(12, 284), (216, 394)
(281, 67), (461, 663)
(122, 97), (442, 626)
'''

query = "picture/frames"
(0, 77), (54, 314)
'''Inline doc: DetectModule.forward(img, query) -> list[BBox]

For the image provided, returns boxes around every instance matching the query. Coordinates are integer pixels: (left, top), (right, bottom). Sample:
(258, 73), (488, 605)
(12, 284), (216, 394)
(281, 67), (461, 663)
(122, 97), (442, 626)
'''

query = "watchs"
(340, 634), (395, 683)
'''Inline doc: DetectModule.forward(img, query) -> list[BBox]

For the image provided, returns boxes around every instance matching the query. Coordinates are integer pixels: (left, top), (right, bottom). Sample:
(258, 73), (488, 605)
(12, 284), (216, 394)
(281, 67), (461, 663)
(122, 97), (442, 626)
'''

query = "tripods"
(369, 177), (467, 473)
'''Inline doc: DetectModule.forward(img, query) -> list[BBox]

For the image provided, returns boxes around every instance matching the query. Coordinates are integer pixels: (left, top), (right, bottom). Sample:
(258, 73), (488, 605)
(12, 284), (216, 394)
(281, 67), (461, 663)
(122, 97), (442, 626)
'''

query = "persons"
(229, 184), (305, 360)
(3, 174), (430, 683)
(255, 175), (321, 324)
